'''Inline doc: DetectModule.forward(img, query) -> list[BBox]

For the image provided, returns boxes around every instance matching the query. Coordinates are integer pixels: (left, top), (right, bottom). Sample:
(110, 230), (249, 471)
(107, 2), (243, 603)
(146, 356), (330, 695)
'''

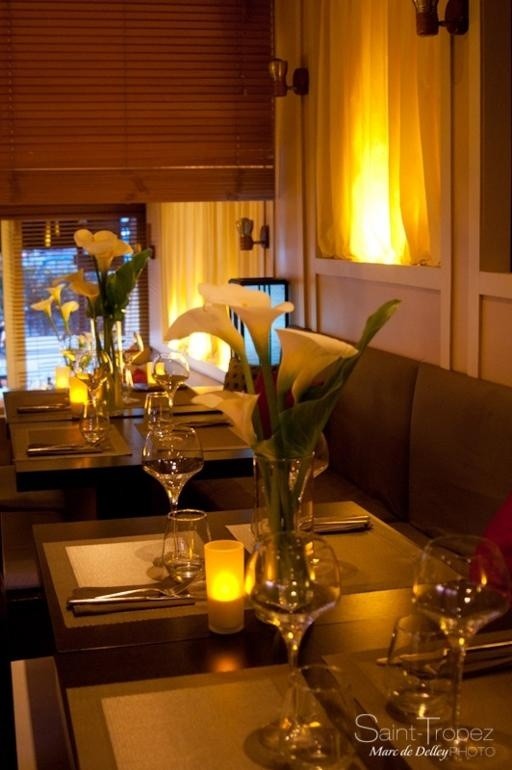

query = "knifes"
(66, 593), (197, 603)
(375, 640), (512, 666)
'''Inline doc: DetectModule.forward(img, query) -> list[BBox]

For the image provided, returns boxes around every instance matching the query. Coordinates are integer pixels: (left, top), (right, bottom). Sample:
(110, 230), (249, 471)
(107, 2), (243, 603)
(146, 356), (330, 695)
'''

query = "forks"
(401, 654), (512, 681)
(94, 579), (197, 599)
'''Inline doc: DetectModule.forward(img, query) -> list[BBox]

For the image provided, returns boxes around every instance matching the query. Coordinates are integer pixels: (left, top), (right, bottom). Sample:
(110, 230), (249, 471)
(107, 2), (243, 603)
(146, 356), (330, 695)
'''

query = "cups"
(408, 533), (496, 769)
(78, 399), (111, 447)
(275, 663), (362, 770)
(247, 534), (342, 751)
(142, 391), (175, 433)
(376, 614), (453, 717)
(159, 505), (213, 583)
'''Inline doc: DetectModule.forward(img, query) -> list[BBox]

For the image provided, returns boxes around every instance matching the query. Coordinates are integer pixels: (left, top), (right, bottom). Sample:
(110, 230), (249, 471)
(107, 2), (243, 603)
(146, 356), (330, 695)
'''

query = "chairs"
(2, 386), (507, 770)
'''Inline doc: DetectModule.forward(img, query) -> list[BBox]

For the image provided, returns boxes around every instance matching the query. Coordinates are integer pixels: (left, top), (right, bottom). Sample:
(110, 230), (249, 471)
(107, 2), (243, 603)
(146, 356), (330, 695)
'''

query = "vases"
(89, 311), (124, 417)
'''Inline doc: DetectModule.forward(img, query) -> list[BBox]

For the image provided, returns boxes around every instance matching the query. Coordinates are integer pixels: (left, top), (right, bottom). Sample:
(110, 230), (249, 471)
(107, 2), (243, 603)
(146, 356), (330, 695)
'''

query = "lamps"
(238, 216), (270, 250)
(269, 58), (311, 97)
(414, 0), (468, 37)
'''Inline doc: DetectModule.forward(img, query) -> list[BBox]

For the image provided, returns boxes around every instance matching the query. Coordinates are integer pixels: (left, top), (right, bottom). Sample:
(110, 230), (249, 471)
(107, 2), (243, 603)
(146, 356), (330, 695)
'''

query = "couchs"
(225, 325), (508, 625)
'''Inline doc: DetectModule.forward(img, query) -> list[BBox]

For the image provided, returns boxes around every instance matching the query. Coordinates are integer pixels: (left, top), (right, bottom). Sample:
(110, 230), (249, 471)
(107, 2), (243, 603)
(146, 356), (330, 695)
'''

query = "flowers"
(164, 279), (404, 590)
(30, 223), (156, 405)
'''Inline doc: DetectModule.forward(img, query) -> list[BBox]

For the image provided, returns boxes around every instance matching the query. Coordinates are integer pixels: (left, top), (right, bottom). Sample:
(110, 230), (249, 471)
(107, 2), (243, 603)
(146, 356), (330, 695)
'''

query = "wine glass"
(138, 424), (206, 560)
(149, 349), (192, 410)
(75, 346), (115, 397)
